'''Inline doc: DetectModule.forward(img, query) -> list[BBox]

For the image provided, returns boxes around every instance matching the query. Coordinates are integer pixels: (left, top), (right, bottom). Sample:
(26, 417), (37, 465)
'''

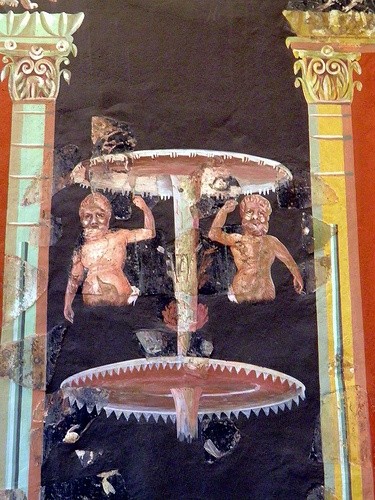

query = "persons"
(63, 192), (158, 325)
(207, 195), (307, 305)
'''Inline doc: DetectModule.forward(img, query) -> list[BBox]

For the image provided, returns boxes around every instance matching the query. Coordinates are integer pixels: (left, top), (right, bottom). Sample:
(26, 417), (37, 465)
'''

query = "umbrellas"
(59, 357), (307, 447)
(71, 149), (294, 360)
